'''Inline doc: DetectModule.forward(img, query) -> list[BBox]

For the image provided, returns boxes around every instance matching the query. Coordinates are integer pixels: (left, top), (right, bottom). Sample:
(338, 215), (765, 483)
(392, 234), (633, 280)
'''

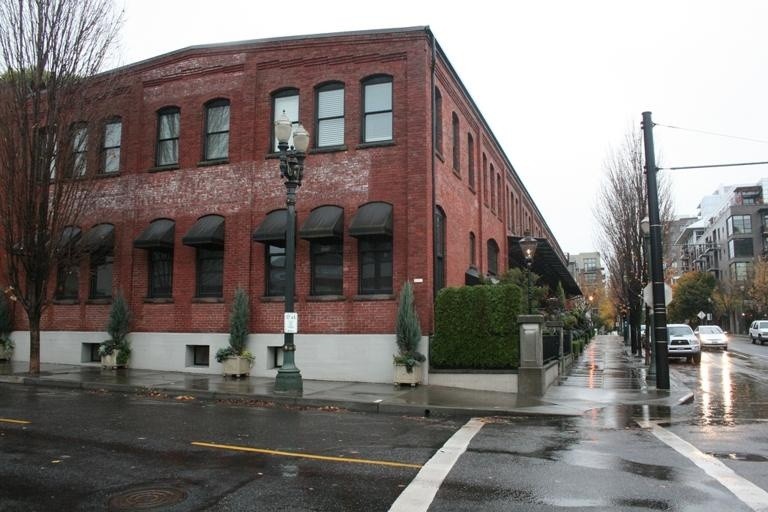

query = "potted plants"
(389, 273), (426, 391)
(97, 288), (133, 369)
(215, 286), (256, 379)
(0, 290), (16, 361)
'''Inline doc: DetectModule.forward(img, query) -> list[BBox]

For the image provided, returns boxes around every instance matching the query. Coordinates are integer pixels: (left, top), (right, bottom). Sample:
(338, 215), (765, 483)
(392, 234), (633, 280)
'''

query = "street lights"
(640, 214), (657, 379)
(275, 109), (310, 397)
(519, 229), (538, 315)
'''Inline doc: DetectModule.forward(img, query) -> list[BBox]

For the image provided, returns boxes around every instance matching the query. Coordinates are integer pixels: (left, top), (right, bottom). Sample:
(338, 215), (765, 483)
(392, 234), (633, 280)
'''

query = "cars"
(749, 320), (768, 345)
(636, 324), (728, 366)
